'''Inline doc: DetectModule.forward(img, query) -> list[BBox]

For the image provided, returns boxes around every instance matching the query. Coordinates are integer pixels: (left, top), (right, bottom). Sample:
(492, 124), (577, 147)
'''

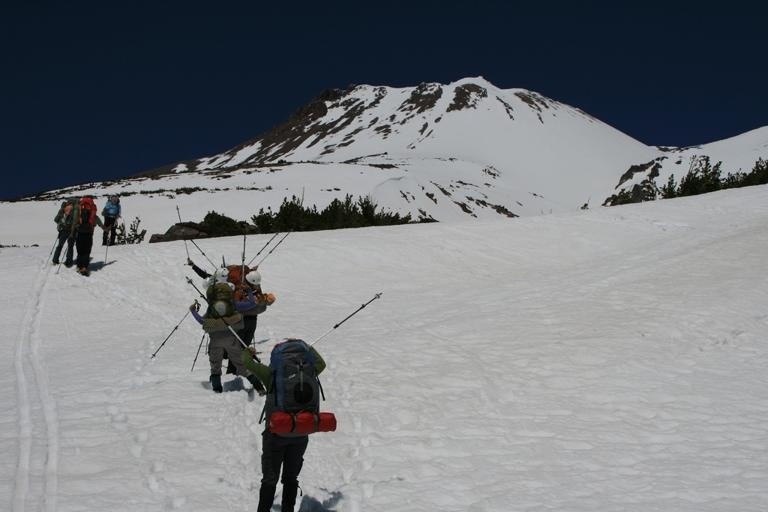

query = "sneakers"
(212, 383), (222, 393)
(80, 267), (88, 277)
(253, 382), (265, 393)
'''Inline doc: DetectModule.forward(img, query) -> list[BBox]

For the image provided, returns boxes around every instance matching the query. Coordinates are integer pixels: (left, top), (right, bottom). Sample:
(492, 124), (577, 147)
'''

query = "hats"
(215, 268), (228, 280)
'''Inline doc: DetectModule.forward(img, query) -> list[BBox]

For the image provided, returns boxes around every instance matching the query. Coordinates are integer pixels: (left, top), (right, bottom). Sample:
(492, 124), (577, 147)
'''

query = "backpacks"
(205, 282), (237, 318)
(61, 201), (73, 227)
(268, 339), (326, 414)
(78, 197), (97, 225)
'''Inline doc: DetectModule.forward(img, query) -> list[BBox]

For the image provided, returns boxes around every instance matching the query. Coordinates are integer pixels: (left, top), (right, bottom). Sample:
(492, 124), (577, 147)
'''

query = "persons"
(186, 256), (258, 360)
(227, 270), (277, 374)
(101, 195), (122, 245)
(52, 196), (75, 267)
(72, 197), (108, 275)
(240, 337), (328, 512)
(189, 282), (270, 397)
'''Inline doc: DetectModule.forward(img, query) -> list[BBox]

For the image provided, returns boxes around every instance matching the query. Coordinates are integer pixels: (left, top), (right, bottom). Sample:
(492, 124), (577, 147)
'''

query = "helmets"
(245, 270), (261, 286)
